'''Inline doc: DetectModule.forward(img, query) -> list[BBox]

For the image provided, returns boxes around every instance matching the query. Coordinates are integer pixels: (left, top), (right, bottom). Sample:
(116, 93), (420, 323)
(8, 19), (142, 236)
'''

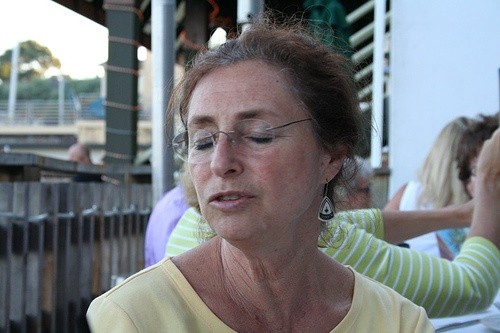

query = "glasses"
(356, 187), (374, 195)
(168, 115), (317, 164)
